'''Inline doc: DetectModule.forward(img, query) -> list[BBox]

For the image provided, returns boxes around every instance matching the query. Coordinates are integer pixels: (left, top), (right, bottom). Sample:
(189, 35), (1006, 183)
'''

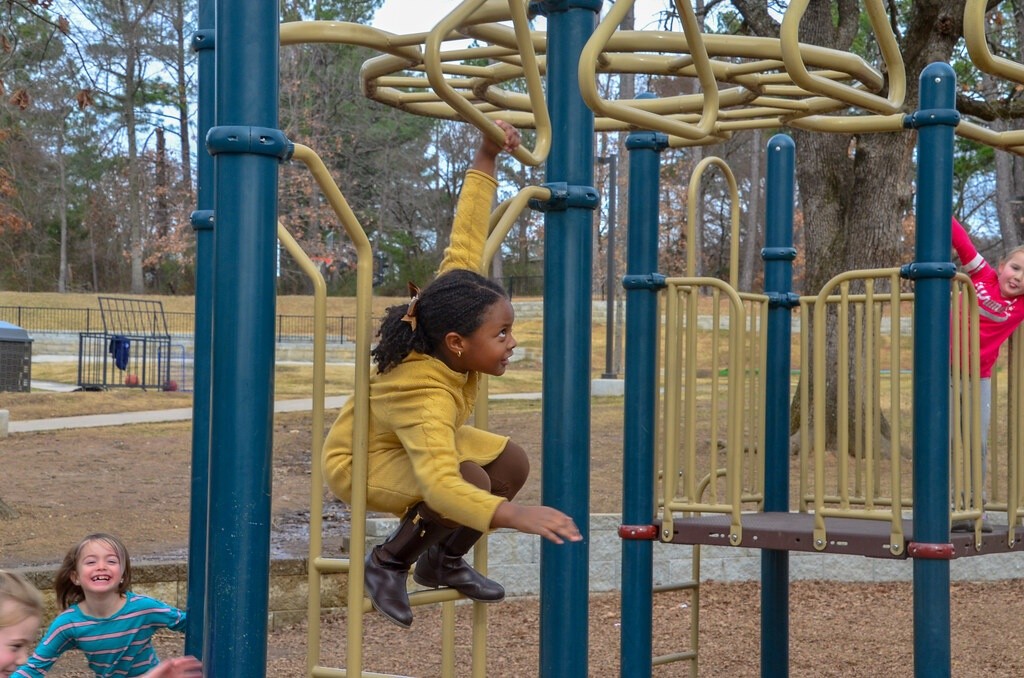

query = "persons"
(321, 120), (584, 628)
(8, 533), (186, 678)
(950, 218), (1024, 532)
(0, 570), (203, 678)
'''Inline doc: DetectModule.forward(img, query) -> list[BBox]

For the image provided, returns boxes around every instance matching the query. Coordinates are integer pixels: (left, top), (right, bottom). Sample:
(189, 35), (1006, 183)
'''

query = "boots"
(365, 502), (462, 629)
(413, 525), (506, 604)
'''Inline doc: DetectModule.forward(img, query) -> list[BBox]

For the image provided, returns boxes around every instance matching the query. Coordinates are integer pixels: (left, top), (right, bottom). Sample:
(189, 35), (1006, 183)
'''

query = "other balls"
(163, 380), (178, 391)
(125, 375), (139, 385)
